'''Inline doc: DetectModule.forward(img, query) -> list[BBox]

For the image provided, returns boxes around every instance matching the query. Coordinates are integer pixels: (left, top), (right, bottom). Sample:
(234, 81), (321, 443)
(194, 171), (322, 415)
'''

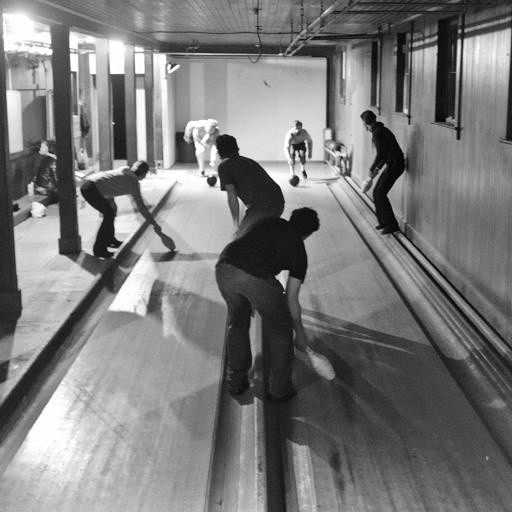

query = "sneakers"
(302, 171), (307, 178)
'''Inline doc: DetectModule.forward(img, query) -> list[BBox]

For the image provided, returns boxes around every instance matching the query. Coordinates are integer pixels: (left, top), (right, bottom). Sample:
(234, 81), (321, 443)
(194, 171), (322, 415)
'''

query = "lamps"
(164, 62), (180, 78)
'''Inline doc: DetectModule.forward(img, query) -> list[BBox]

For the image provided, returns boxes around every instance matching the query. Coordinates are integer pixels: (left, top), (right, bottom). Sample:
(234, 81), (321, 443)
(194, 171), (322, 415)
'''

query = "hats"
(205, 119), (218, 133)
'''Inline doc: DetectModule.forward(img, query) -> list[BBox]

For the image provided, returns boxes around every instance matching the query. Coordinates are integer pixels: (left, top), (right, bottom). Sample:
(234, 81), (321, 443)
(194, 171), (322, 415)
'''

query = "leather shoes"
(108, 237), (122, 247)
(382, 227), (399, 234)
(95, 250), (113, 258)
(376, 225), (385, 230)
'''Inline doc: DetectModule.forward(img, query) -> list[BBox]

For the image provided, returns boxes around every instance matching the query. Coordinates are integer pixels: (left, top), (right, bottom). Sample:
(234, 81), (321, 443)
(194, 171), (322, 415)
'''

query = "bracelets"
(375, 165), (381, 172)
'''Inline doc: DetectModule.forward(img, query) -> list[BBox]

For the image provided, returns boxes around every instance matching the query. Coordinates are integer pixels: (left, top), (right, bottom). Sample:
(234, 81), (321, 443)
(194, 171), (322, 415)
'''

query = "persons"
(34, 156), (78, 204)
(215, 206), (321, 405)
(183, 119), (220, 176)
(285, 119), (313, 179)
(79, 159), (162, 259)
(215, 133), (286, 240)
(31, 140), (57, 182)
(359, 109), (405, 235)
